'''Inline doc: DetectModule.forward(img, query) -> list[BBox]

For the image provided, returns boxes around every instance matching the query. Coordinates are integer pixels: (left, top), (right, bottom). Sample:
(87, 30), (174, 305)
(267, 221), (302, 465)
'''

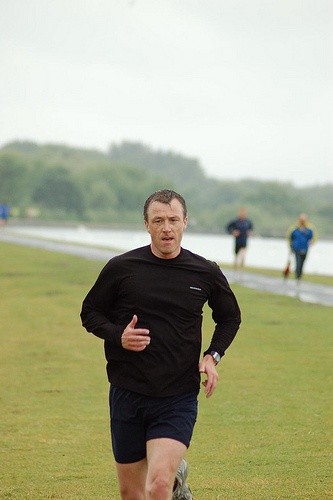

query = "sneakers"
(175, 459), (193, 500)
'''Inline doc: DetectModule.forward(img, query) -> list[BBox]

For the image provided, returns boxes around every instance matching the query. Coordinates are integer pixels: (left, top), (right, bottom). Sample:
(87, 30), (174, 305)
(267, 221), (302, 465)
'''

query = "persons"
(80, 190), (241, 500)
(0, 200), (9, 226)
(285, 214), (318, 281)
(226, 209), (253, 268)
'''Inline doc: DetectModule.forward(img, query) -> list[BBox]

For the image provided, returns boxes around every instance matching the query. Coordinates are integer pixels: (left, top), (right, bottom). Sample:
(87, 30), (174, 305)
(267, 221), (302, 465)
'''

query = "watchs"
(209, 352), (220, 365)
